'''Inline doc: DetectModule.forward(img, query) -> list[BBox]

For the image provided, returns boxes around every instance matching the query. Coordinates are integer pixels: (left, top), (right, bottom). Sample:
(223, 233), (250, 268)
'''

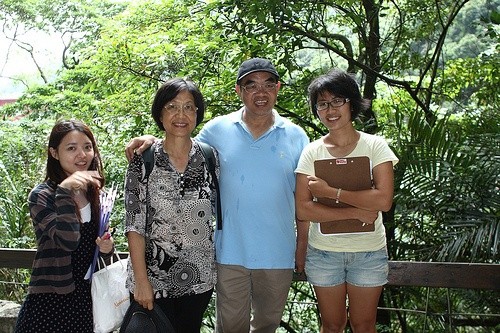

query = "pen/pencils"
(103, 227), (116, 239)
(361, 222), (366, 227)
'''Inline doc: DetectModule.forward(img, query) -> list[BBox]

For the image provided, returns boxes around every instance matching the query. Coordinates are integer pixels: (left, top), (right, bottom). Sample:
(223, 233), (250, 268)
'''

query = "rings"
(75, 184), (81, 188)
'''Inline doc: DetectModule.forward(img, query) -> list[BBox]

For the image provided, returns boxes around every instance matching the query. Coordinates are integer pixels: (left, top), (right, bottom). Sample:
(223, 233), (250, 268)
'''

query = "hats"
(236, 57), (280, 82)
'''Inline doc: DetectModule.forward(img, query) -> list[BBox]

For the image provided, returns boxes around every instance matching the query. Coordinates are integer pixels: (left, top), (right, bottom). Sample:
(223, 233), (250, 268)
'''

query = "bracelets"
(334, 187), (342, 203)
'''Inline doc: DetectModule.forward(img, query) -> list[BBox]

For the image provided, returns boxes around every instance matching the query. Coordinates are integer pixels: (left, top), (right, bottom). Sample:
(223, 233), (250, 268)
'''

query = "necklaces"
(71, 188), (80, 195)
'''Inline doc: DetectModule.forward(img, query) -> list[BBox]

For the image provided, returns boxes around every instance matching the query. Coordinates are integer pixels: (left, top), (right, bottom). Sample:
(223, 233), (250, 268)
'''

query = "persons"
(294, 69), (400, 333)
(126, 57), (310, 333)
(15, 118), (116, 333)
(124, 77), (221, 333)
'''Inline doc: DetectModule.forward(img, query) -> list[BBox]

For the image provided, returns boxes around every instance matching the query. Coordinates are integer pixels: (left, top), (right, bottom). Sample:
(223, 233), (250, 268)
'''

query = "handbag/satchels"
(91, 248), (130, 333)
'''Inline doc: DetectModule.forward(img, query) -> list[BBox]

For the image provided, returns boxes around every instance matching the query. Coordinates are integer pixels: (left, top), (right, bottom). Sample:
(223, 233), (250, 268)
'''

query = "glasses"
(240, 83), (278, 94)
(163, 102), (198, 116)
(314, 97), (350, 111)
